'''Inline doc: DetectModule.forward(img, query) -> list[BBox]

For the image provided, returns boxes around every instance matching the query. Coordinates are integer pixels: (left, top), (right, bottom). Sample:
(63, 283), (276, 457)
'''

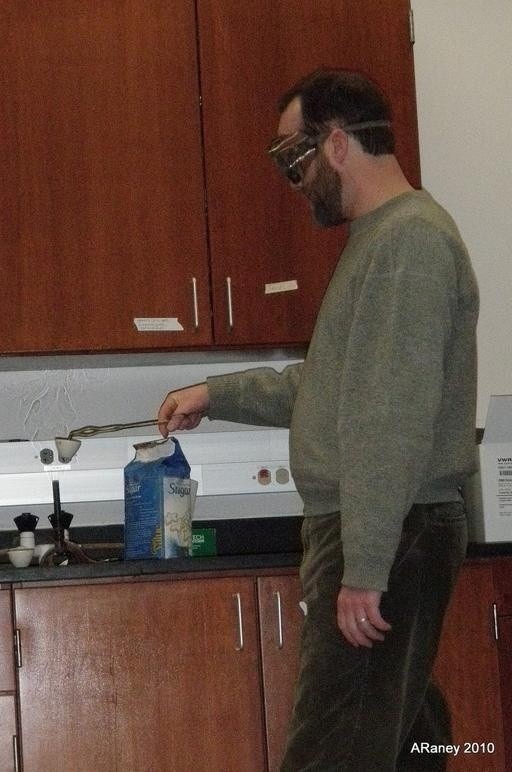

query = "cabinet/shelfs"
(0, 1), (420, 357)
(5, 556), (510, 771)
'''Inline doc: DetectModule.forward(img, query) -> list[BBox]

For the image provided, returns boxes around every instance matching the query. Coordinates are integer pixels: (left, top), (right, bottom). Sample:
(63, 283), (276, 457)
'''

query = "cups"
(6, 549), (35, 568)
(55, 437), (83, 457)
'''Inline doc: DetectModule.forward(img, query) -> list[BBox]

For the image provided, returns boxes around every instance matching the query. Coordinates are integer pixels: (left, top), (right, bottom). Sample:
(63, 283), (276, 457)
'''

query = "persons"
(156, 68), (480, 772)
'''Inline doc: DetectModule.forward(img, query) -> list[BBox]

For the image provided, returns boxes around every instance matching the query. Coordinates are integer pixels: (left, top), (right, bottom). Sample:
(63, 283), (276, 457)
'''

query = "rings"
(355, 616), (368, 625)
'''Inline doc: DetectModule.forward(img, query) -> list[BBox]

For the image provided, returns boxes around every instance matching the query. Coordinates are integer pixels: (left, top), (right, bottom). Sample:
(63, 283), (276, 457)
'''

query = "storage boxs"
(464, 390), (511, 548)
